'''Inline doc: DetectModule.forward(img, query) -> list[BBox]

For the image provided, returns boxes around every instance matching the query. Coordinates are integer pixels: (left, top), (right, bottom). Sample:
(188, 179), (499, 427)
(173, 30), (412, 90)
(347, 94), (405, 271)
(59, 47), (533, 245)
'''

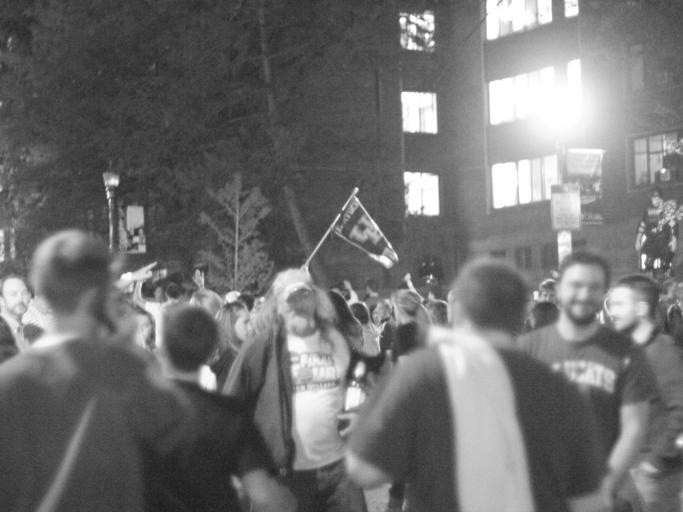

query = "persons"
(155, 305), (297, 512)
(343, 258), (604, 512)
(529, 301), (559, 330)
(608, 274), (683, 511)
(389, 290), (432, 498)
(325, 273), (449, 358)
(115, 261), (262, 383)
(537, 278), (557, 305)
(0, 273), (44, 363)
(0, 228), (190, 511)
(516, 251), (651, 511)
(221, 268), (367, 511)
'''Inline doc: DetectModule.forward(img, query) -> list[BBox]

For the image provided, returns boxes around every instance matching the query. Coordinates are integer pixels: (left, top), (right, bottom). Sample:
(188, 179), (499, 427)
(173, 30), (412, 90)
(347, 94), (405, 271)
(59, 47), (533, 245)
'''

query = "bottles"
(336, 354), (367, 435)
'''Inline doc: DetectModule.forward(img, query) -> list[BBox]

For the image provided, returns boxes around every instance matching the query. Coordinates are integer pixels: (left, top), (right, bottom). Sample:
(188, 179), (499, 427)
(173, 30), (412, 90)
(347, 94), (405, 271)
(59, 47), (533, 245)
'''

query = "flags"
(302, 186), (401, 270)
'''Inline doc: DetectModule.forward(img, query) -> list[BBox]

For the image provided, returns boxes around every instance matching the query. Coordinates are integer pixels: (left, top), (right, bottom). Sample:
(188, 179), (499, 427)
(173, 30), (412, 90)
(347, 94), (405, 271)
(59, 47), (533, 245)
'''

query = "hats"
(272, 269), (314, 302)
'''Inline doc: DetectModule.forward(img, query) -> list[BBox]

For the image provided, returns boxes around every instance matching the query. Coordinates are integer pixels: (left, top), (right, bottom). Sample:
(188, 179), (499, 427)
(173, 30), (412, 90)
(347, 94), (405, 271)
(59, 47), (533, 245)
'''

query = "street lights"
(102, 160), (122, 254)
